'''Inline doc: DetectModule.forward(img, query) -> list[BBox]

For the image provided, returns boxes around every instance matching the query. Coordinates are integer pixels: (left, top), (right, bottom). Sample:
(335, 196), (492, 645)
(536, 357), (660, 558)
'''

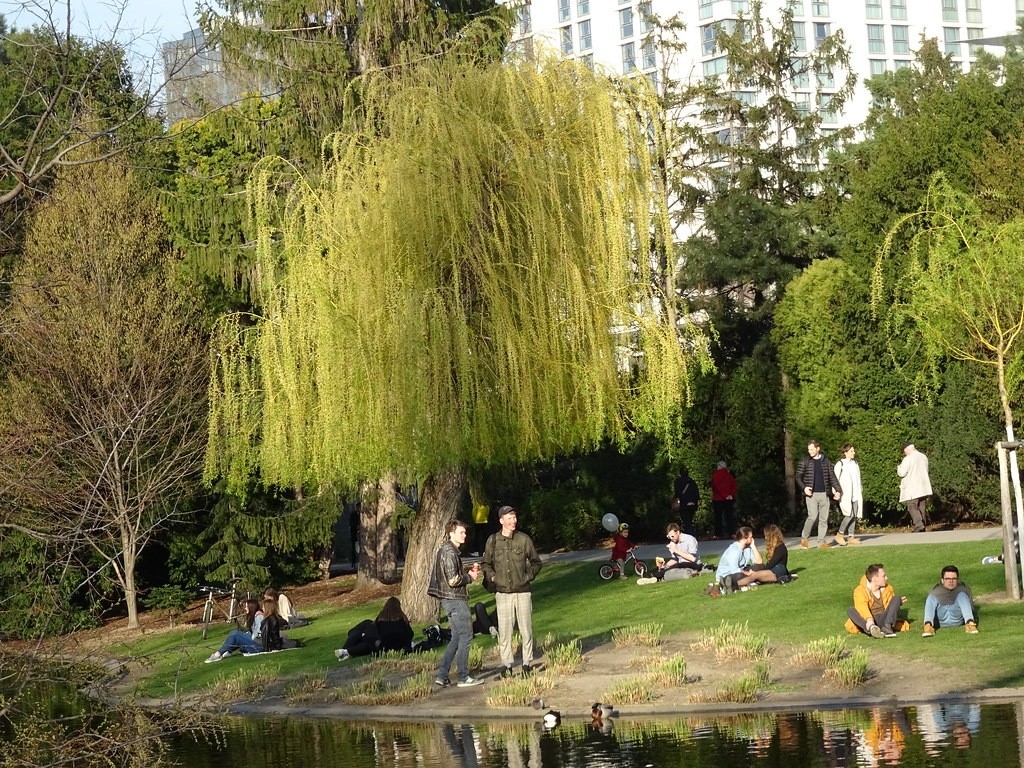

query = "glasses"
(666, 532), (677, 539)
(943, 577), (957, 581)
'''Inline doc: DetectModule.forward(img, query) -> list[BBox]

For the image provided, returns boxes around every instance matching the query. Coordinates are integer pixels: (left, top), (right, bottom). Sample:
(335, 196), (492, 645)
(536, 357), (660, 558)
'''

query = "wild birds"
(542, 710), (560, 721)
(591, 703), (620, 719)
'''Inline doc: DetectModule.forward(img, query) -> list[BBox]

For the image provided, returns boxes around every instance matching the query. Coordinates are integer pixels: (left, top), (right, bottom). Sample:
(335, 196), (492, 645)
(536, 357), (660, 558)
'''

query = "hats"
(498, 505), (517, 518)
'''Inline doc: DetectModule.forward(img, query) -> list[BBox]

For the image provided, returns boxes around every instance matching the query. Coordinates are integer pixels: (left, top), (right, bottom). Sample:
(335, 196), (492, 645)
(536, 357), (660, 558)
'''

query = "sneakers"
(435, 677), (451, 687)
(922, 623), (935, 638)
(965, 622), (979, 634)
(457, 676), (485, 687)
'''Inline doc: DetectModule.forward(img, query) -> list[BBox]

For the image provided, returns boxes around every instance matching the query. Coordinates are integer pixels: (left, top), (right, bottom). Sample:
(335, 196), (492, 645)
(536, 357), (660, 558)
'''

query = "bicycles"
(599, 546), (649, 581)
(195, 579), (248, 637)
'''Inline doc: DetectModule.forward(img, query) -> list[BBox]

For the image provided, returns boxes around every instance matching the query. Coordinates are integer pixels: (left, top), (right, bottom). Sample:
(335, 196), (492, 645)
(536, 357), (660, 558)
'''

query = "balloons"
(602, 513), (620, 532)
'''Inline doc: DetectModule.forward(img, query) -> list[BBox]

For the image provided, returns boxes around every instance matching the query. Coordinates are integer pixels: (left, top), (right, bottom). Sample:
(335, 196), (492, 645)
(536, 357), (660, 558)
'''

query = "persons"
(611, 523), (638, 580)
(674, 467), (699, 542)
(716, 525), (790, 594)
(844, 564), (909, 639)
(481, 506), (543, 682)
(637, 522), (701, 586)
(711, 460), (737, 540)
(350, 502), (361, 569)
(469, 496), (490, 556)
(795, 440), (842, 549)
(204, 587), (297, 664)
(427, 520), (485, 687)
(335, 597), (519, 661)
(396, 491), (418, 562)
(831, 443), (864, 545)
(897, 442), (933, 532)
(921, 566), (979, 637)
(982, 499), (1024, 565)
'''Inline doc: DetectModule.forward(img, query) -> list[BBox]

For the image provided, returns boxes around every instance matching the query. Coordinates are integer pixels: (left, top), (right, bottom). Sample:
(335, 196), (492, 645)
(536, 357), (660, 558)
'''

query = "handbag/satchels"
(482, 573), (496, 594)
(288, 616), (309, 630)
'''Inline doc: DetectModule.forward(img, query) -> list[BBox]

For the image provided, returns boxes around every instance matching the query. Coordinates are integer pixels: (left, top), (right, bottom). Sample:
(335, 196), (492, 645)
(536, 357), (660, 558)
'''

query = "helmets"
(618, 523), (629, 532)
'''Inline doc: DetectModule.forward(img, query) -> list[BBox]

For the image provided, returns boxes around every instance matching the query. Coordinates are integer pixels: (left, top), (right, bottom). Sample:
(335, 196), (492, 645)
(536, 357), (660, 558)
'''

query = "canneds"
(472, 563), (479, 572)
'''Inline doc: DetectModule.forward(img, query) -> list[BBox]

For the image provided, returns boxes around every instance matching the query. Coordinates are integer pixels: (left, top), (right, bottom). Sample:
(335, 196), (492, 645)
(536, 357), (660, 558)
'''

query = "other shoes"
(800, 539), (834, 550)
(982, 556), (998, 565)
(471, 551), (479, 557)
(500, 666), (513, 679)
(522, 665), (530, 678)
(620, 575), (628, 579)
(221, 651), (232, 657)
(489, 626), (498, 638)
(204, 654), (222, 664)
(869, 624), (897, 639)
(335, 648), (345, 658)
(701, 574), (738, 595)
(641, 568), (651, 578)
(911, 525), (926, 533)
(637, 576), (657, 585)
(834, 533), (861, 546)
